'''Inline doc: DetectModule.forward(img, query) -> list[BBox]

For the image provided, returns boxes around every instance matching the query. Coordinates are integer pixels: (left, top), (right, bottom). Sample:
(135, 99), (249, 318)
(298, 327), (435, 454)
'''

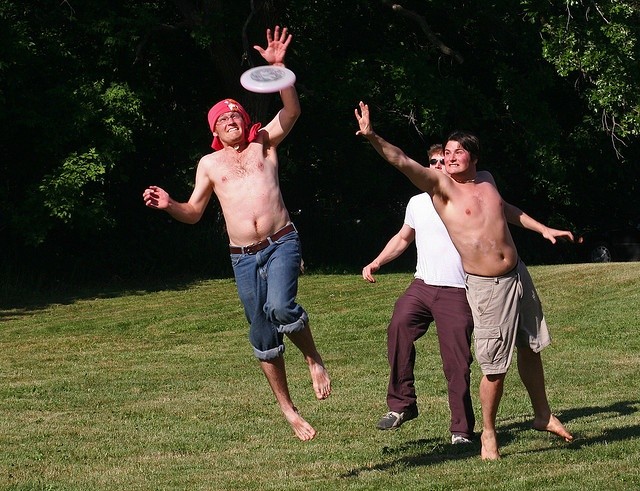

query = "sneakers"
(451, 435), (473, 446)
(376, 406), (419, 430)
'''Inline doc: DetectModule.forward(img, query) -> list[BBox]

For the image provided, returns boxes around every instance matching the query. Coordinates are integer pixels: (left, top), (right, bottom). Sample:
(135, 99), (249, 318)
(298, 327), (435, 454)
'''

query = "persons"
(143, 24), (333, 443)
(354, 98), (578, 462)
(362, 141), (475, 445)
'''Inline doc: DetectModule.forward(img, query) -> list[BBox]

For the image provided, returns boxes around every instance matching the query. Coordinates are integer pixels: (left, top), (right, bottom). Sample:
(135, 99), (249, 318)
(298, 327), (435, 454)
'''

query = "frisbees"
(240, 66), (296, 93)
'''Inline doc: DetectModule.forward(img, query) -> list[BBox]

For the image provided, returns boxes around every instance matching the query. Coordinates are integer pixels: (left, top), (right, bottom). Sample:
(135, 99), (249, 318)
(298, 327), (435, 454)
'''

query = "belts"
(229, 224), (295, 254)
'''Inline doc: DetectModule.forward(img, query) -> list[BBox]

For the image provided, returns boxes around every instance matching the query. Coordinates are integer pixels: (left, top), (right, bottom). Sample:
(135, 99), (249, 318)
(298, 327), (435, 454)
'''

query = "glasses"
(431, 158), (444, 165)
